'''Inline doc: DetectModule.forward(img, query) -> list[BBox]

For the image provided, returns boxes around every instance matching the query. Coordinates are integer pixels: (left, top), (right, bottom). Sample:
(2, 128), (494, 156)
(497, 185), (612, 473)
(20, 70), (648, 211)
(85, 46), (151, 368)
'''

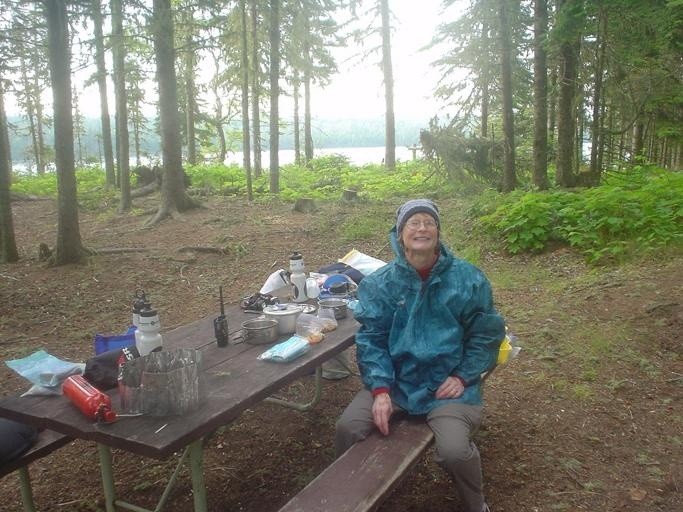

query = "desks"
(1, 292), (365, 511)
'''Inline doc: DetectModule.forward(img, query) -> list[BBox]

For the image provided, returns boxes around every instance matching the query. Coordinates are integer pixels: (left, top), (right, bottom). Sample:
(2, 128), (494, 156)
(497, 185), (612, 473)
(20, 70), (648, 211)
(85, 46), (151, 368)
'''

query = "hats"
(394, 199), (440, 239)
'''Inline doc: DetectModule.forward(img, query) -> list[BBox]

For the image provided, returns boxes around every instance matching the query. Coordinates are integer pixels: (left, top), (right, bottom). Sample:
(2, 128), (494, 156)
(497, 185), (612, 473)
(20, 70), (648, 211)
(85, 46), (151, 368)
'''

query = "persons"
(334, 199), (504, 512)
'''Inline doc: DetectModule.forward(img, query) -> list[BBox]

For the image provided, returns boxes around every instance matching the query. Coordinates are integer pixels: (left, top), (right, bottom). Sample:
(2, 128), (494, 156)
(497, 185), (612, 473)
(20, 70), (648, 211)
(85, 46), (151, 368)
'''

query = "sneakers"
(481, 503), (490, 512)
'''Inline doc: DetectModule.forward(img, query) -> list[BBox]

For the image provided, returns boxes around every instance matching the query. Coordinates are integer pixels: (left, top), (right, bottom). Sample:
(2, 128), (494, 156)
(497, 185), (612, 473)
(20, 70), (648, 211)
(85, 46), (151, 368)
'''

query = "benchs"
(276, 365), (501, 509)
(1, 418), (79, 511)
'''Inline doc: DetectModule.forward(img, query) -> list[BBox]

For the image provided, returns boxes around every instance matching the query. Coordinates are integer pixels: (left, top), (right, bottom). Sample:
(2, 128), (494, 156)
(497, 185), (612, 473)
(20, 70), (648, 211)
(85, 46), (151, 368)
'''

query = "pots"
(262, 303), (301, 337)
(316, 299), (346, 319)
(231, 318), (279, 348)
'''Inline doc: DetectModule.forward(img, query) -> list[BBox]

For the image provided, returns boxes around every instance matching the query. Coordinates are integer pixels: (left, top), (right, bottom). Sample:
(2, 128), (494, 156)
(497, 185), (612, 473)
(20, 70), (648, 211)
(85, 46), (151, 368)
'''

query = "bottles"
(60, 373), (117, 424)
(289, 249), (309, 303)
(136, 300), (162, 359)
(131, 288), (149, 328)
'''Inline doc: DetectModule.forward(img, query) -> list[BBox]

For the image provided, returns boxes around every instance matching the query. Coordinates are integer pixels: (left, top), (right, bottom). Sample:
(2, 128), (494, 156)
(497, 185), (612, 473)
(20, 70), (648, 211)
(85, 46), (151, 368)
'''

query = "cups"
(305, 278), (319, 297)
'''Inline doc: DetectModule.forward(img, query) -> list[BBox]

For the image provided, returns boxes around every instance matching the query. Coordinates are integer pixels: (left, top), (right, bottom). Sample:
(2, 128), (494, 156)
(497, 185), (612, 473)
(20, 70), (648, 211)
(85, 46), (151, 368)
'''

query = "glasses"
(405, 220), (438, 230)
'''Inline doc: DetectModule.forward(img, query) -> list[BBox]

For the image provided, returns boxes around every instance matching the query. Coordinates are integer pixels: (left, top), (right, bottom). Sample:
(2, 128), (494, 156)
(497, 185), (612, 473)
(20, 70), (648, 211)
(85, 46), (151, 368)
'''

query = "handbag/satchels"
(2, 325), (140, 400)
